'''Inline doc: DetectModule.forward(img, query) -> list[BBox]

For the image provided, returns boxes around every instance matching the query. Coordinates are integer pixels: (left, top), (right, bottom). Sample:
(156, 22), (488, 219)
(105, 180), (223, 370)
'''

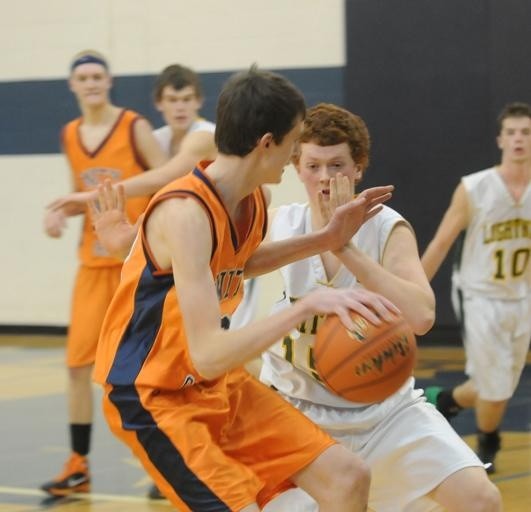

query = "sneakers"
(475, 430), (501, 473)
(423, 386), (444, 406)
(40, 452), (91, 497)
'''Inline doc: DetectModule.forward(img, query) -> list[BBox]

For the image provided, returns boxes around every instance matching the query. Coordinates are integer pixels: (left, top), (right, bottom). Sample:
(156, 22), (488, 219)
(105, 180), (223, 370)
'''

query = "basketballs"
(313, 305), (418, 405)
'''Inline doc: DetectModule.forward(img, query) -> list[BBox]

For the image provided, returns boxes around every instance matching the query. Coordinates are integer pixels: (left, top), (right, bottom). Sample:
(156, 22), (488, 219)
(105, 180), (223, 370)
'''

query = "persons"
(420, 101), (531, 473)
(41, 50), (172, 499)
(87, 103), (501, 512)
(46, 65), (216, 217)
(92, 62), (401, 512)
